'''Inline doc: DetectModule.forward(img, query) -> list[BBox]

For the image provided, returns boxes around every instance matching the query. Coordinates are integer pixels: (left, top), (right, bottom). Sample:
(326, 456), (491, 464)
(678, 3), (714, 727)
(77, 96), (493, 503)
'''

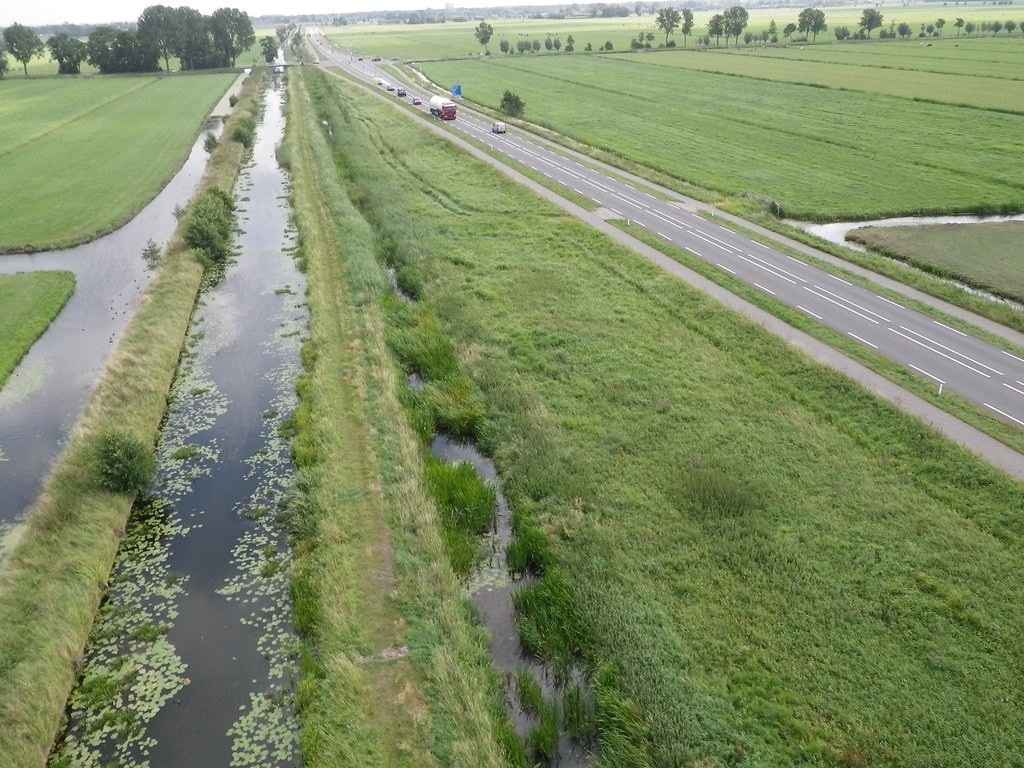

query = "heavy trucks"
(430, 95), (457, 121)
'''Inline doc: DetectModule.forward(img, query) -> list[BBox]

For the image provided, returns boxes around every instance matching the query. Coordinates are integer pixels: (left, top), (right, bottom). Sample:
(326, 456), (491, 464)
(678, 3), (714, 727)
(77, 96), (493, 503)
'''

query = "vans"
(397, 87), (407, 96)
(374, 77), (382, 85)
(492, 122), (507, 133)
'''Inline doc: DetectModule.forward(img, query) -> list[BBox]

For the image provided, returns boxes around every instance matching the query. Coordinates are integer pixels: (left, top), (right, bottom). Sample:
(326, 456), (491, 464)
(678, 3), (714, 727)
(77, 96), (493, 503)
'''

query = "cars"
(390, 56), (400, 61)
(387, 85), (395, 90)
(371, 56), (381, 61)
(413, 96), (422, 105)
